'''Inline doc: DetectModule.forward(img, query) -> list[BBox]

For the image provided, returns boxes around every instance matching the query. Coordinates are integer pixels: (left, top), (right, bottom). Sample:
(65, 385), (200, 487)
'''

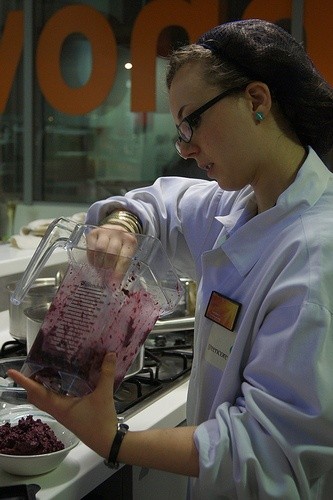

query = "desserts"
(0, 415), (64, 455)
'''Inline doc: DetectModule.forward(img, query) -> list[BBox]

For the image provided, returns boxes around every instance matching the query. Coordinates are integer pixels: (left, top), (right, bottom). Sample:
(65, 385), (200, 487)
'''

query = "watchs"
(104, 424), (130, 470)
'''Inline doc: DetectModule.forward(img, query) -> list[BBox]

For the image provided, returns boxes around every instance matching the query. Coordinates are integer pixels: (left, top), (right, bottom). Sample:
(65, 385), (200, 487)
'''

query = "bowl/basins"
(31, 219), (55, 236)
(0, 411), (79, 476)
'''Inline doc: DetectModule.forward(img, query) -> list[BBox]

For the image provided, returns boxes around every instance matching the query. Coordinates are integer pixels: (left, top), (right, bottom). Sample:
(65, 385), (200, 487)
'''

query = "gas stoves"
(1, 337), (195, 423)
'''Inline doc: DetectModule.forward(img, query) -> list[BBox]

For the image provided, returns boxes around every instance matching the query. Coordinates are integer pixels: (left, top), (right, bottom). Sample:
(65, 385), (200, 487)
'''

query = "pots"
(4, 277), (57, 340)
(55, 270), (198, 332)
(24, 303), (144, 379)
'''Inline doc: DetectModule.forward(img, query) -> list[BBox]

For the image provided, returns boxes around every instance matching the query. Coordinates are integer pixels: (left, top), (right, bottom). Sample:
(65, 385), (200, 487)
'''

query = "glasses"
(174, 83), (254, 161)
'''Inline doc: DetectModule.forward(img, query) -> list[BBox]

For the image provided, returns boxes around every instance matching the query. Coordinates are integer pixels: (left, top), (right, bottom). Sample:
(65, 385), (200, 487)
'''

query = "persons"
(5, 17), (333, 500)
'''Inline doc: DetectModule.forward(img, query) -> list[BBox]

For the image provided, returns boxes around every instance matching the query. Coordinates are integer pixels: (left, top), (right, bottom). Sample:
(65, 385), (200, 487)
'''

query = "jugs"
(8, 216), (185, 403)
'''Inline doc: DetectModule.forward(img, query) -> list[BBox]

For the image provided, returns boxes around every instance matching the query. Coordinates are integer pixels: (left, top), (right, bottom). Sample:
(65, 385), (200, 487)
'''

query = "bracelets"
(98, 208), (144, 235)
